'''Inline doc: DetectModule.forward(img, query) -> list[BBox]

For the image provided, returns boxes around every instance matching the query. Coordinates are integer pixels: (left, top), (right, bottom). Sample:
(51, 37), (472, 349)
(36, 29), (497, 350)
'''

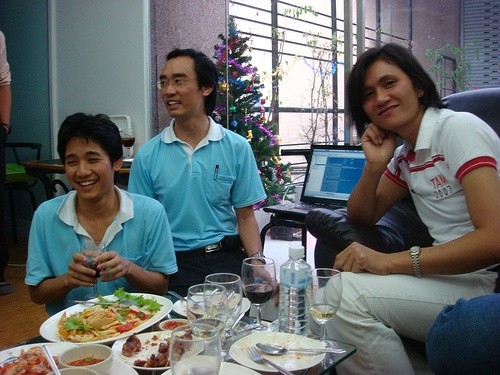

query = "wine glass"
(80, 241), (108, 300)
(240, 256), (277, 331)
(305, 268), (343, 348)
(119, 128), (135, 159)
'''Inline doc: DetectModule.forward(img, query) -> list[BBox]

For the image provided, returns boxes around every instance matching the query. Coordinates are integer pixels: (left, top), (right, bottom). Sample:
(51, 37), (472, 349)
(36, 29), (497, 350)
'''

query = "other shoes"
(0, 281), (17, 295)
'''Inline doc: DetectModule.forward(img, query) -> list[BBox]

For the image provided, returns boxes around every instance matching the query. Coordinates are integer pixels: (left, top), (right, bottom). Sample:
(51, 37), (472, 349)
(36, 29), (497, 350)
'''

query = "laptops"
(263, 144), (367, 216)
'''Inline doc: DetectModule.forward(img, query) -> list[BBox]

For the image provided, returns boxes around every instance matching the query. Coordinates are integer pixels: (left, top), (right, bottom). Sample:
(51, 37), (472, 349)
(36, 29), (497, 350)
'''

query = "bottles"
(278, 245), (311, 338)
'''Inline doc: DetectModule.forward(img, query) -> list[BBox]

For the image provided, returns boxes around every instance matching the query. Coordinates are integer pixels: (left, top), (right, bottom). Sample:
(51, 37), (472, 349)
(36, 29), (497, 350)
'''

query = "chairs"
(3, 142), (41, 244)
(260, 214), (315, 270)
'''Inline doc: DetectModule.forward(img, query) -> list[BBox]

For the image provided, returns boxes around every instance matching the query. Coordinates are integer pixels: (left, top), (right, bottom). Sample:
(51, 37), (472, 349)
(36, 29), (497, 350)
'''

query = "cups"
(57, 343), (113, 375)
(45, 366), (98, 375)
(168, 273), (243, 375)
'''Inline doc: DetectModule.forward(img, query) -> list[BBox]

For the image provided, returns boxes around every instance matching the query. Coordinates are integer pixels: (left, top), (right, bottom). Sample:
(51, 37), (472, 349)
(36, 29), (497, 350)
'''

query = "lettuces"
(64, 287), (163, 331)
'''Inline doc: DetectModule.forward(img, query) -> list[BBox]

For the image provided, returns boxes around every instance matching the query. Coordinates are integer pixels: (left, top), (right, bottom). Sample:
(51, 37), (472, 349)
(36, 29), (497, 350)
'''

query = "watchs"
(250, 253), (266, 263)
(410, 246), (422, 277)
(2, 123), (11, 135)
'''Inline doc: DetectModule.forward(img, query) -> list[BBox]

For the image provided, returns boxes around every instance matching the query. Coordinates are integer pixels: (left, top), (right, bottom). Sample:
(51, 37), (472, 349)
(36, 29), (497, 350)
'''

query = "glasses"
(156, 77), (198, 90)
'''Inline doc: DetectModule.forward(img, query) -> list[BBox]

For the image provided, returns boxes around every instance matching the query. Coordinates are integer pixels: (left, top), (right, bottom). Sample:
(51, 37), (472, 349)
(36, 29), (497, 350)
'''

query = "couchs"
(304, 89), (500, 284)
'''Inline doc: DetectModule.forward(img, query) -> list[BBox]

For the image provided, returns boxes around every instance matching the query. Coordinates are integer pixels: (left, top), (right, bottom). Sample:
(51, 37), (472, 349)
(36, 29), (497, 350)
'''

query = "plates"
(173, 292), (250, 321)
(39, 293), (170, 346)
(160, 361), (261, 375)
(229, 332), (327, 372)
(0, 342), (140, 375)
(111, 331), (204, 370)
(159, 318), (188, 330)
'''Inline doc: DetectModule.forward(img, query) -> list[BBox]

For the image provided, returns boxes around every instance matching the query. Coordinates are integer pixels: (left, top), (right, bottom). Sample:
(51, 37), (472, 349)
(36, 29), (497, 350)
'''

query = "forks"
(246, 345), (293, 375)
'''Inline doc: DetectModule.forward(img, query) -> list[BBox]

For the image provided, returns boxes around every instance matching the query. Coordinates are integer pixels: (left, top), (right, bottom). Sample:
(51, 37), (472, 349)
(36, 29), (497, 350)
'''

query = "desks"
(23, 159), (131, 197)
(0, 310), (358, 375)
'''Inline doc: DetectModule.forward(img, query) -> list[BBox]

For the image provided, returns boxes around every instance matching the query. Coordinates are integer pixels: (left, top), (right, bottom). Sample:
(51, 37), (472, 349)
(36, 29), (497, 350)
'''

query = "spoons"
(256, 343), (348, 355)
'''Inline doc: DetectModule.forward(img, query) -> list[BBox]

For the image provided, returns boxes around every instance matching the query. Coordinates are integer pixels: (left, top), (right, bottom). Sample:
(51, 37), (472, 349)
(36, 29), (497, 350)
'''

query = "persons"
(127, 50), (280, 318)
(24, 112), (178, 317)
(308, 42), (500, 375)
(0, 31), (16, 295)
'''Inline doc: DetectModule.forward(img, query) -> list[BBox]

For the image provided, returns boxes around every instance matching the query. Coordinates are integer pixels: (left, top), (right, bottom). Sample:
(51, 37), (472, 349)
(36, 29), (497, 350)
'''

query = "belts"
(175, 235), (241, 258)
